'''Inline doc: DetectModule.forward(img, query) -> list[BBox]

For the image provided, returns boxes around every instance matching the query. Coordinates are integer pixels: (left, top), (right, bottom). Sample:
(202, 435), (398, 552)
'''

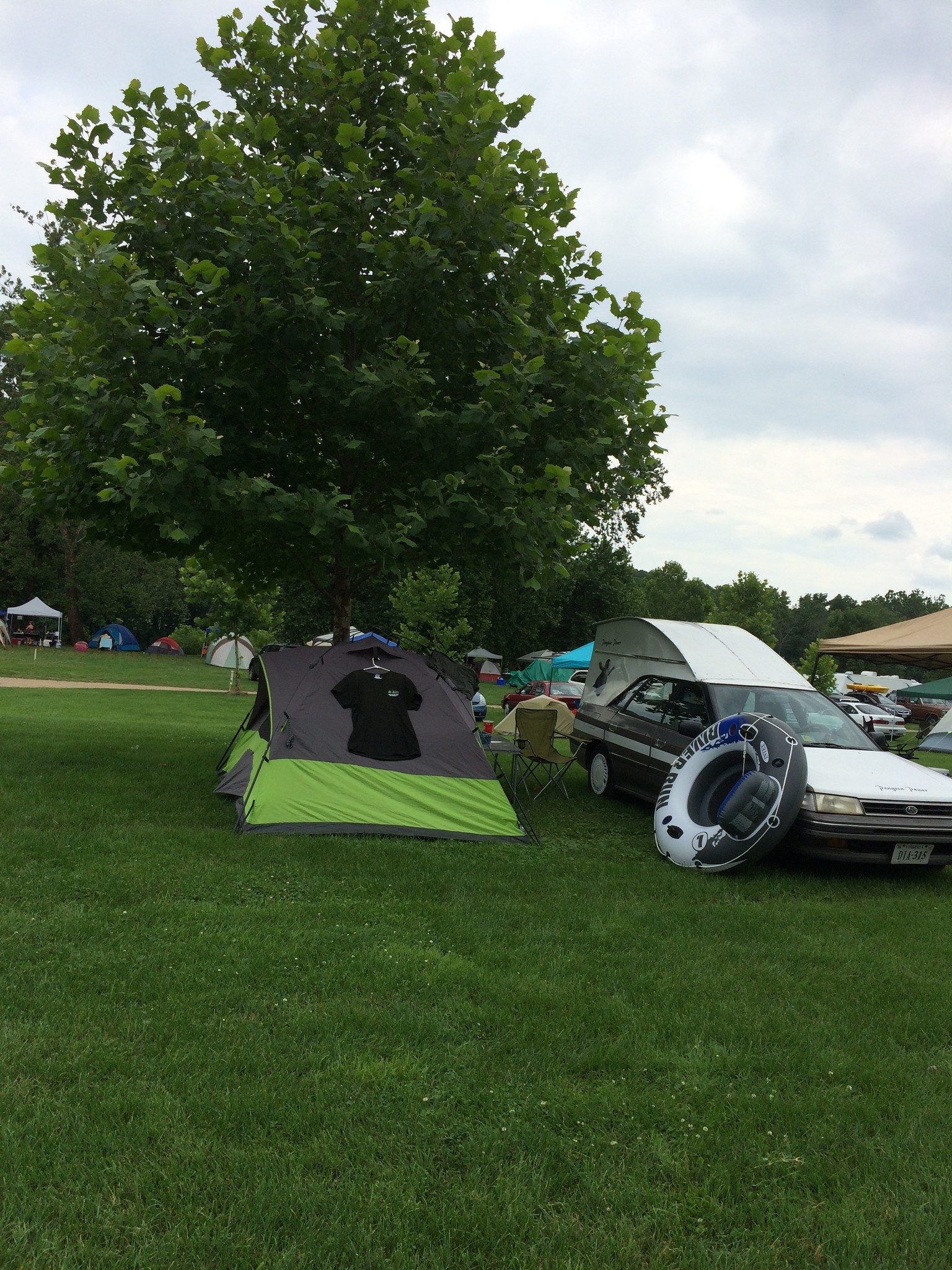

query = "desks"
(483, 735), (521, 805)
(44, 635), (58, 640)
(13, 632), (40, 637)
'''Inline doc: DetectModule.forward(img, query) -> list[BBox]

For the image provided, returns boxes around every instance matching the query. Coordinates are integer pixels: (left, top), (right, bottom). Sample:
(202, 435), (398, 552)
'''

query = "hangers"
(363, 657), (390, 672)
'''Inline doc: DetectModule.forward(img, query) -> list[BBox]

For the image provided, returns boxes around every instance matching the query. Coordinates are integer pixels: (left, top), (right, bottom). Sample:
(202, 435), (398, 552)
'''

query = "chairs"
(881, 740), (920, 760)
(917, 722), (931, 745)
(754, 690), (787, 723)
(512, 708), (592, 800)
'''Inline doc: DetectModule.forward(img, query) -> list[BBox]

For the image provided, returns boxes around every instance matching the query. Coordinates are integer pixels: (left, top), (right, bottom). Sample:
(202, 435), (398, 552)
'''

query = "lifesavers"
(653, 712), (809, 877)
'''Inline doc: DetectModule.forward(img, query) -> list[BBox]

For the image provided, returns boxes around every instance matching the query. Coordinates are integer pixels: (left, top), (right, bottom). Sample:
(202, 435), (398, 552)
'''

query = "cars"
(785, 670), (952, 741)
(570, 616), (952, 872)
(501, 680), (583, 715)
(567, 670), (588, 691)
(472, 691), (487, 722)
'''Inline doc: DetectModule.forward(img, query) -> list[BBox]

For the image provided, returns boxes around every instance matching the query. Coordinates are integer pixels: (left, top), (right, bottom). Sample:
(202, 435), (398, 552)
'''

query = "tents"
(88, 624), (141, 652)
(0, 611), (6, 624)
(4, 597), (62, 648)
(811, 607), (952, 742)
(201, 626), (594, 844)
(144, 637), (185, 656)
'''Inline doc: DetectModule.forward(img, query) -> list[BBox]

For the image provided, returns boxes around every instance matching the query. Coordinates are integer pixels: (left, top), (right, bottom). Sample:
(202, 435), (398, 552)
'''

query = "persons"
(18, 629), (24, 646)
(26, 621), (34, 644)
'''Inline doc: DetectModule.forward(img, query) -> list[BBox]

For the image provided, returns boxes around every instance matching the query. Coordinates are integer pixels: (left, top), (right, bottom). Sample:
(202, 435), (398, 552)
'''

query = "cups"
(483, 720), (494, 734)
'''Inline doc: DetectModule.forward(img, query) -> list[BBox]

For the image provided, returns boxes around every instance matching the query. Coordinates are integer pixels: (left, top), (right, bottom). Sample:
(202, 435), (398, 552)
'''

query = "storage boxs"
(43, 640), (52, 647)
(12, 638), (24, 644)
(54, 640), (62, 646)
